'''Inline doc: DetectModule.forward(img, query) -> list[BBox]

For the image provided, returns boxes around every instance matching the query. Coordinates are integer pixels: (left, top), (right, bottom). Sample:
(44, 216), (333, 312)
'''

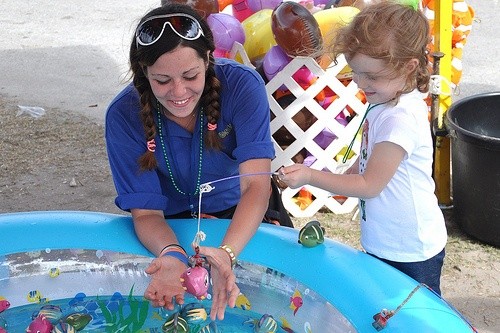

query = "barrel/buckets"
(446, 90), (500, 249)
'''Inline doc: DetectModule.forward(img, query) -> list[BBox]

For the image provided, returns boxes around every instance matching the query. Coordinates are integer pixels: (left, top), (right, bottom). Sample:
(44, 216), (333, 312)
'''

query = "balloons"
(161, 0), (474, 210)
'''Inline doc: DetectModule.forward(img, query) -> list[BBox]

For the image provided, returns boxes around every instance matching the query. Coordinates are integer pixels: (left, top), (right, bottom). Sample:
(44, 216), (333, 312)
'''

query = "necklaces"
(155, 99), (203, 222)
(341, 99), (393, 163)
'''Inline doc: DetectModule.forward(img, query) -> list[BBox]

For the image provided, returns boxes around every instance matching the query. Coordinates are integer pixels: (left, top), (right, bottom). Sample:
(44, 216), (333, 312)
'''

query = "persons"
(277, 0), (448, 299)
(104, 5), (294, 321)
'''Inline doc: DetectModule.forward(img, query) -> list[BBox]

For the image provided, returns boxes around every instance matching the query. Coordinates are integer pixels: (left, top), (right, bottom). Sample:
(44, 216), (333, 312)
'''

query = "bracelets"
(159, 244), (189, 264)
(218, 245), (237, 271)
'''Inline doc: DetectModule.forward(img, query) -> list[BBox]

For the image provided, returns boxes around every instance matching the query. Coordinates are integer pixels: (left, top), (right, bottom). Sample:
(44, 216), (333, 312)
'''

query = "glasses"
(136, 13), (205, 49)
